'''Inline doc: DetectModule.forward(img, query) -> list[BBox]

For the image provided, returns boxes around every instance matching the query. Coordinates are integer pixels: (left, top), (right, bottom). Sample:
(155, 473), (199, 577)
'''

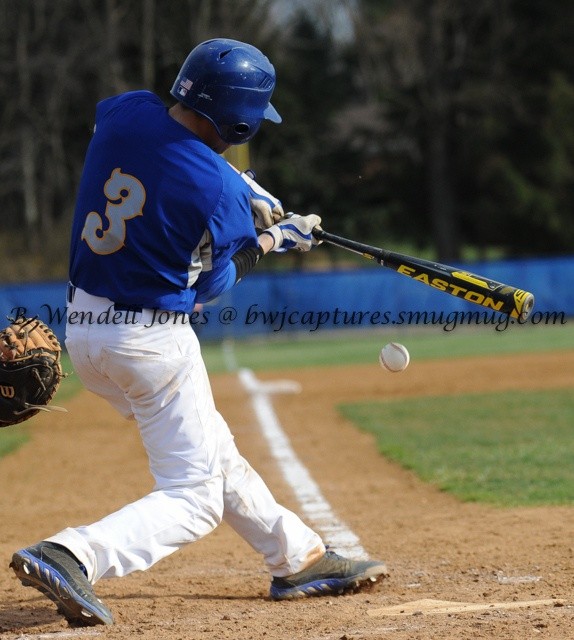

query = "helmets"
(169, 38), (283, 145)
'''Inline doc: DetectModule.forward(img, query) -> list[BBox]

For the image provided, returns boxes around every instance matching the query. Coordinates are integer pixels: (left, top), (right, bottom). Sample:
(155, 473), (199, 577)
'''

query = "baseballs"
(379, 343), (409, 373)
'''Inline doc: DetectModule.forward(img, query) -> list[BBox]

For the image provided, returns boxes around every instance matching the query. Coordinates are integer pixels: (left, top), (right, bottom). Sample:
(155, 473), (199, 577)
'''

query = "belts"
(67, 283), (142, 313)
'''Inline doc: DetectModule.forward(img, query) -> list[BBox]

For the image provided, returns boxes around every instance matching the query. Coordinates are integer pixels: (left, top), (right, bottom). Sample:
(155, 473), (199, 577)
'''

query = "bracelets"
(231, 240), (264, 286)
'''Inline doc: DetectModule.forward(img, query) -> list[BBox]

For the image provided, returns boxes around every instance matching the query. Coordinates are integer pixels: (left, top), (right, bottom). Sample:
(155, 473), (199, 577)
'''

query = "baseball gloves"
(0, 312), (70, 428)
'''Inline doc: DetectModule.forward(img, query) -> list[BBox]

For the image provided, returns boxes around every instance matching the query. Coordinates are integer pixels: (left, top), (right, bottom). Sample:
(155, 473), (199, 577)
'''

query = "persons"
(9, 37), (390, 629)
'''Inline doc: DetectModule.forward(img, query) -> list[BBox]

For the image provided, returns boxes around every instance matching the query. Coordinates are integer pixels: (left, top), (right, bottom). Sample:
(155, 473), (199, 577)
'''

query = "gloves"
(239, 168), (285, 230)
(258, 212), (324, 256)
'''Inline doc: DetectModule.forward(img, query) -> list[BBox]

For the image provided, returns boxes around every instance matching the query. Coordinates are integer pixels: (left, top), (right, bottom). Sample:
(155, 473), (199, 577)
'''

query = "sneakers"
(271, 551), (389, 600)
(12, 542), (113, 626)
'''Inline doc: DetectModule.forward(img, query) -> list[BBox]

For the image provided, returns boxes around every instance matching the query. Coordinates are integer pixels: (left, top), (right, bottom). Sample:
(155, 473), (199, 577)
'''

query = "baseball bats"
(272, 211), (534, 322)
